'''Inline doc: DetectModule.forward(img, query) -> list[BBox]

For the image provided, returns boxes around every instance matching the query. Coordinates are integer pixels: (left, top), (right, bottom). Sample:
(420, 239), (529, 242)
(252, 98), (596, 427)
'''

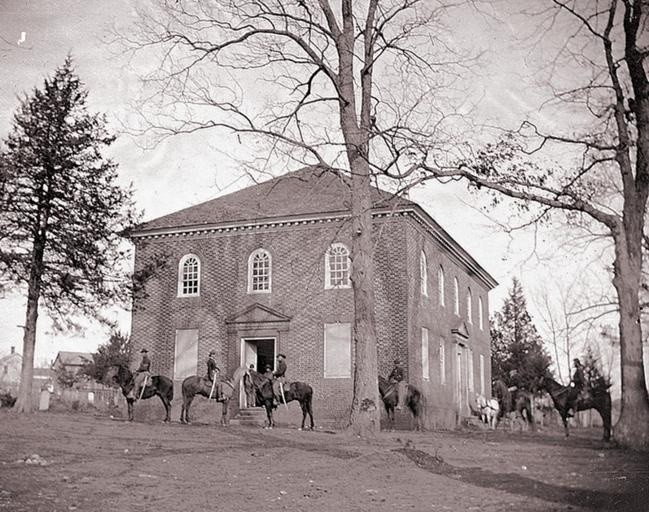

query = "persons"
(563, 357), (588, 415)
(272, 352), (287, 406)
(389, 358), (406, 411)
(206, 349), (227, 402)
(507, 368), (524, 412)
(244, 364), (257, 408)
(260, 363), (273, 407)
(129, 349), (151, 401)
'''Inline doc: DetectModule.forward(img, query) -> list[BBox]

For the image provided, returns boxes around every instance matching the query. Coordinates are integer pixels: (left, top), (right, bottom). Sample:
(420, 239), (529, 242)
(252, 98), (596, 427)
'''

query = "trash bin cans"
(39, 388), (50, 411)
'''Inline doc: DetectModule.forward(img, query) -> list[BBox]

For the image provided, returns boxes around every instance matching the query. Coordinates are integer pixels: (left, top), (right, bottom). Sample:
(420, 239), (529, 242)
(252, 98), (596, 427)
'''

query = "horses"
(243, 369), (315, 432)
(110, 364), (173, 424)
(180, 366), (248, 426)
(492, 378), (533, 430)
(474, 392), (500, 430)
(529, 374), (612, 442)
(377, 375), (426, 433)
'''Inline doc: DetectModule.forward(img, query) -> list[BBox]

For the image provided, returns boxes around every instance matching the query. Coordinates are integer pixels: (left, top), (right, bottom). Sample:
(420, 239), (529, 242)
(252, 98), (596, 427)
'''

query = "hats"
(394, 360), (400, 364)
(140, 348), (148, 353)
(276, 354), (286, 358)
(210, 350), (216, 354)
(264, 363), (272, 369)
